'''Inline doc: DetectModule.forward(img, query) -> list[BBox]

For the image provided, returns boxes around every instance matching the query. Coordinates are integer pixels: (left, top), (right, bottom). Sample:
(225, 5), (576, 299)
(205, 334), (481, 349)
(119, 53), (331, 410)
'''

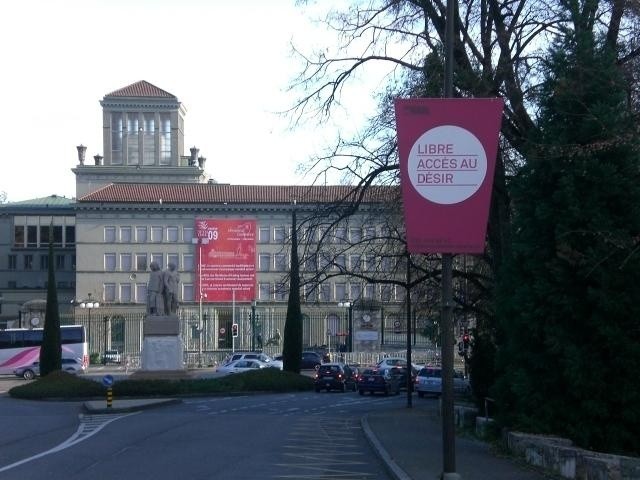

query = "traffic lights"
(462, 335), (470, 350)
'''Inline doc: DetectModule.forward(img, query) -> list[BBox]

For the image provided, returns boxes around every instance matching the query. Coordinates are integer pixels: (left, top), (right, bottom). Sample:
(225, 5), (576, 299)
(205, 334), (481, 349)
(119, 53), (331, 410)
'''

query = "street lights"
(335, 293), (359, 352)
(249, 297), (258, 351)
(78, 292), (99, 359)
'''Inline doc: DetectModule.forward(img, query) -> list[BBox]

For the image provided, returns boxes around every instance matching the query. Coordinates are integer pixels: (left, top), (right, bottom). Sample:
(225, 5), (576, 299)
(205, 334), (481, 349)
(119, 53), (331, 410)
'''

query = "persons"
(264, 328), (283, 347)
(257, 332), (263, 348)
(146, 261), (164, 318)
(163, 262), (180, 317)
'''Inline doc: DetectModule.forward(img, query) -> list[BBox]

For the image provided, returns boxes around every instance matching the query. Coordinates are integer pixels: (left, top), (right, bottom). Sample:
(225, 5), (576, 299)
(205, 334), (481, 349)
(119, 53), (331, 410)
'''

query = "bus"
(0, 323), (87, 379)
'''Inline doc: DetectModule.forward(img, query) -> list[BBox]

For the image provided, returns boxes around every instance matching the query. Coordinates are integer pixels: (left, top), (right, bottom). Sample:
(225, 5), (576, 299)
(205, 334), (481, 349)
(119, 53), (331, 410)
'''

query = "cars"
(102, 349), (121, 364)
(214, 349), (468, 398)
(12, 357), (85, 381)
